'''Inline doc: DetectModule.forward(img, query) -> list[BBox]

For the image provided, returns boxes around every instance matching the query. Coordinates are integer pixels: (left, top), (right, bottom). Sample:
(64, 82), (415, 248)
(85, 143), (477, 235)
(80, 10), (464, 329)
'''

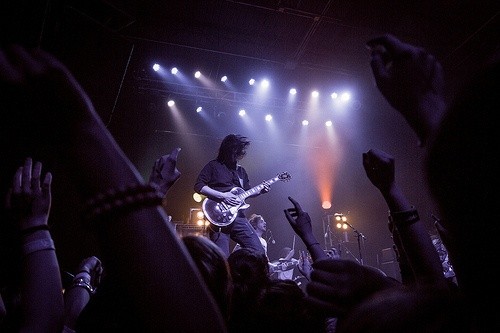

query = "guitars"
(202, 170), (292, 227)
(274, 261), (298, 270)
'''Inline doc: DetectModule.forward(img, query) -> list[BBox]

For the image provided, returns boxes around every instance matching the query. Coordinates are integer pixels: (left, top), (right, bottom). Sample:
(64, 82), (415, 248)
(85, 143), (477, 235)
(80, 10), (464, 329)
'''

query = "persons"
(0, 34), (500, 333)
(194, 134), (272, 259)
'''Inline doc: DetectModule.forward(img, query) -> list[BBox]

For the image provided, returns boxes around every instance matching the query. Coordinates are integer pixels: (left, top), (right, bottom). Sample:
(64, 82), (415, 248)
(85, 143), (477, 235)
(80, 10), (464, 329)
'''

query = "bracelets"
(80, 183), (167, 229)
(19, 239), (56, 257)
(18, 225), (51, 236)
(390, 205), (420, 226)
(68, 277), (96, 297)
(73, 270), (93, 277)
(307, 242), (320, 249)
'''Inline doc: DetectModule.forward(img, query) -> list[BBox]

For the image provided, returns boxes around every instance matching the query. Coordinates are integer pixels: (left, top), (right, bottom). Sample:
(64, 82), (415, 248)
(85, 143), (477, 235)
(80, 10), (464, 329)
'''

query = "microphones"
(269, 230), (275, 245)
(325, 225), (330, 239)
(334, 213), (343, 217)
